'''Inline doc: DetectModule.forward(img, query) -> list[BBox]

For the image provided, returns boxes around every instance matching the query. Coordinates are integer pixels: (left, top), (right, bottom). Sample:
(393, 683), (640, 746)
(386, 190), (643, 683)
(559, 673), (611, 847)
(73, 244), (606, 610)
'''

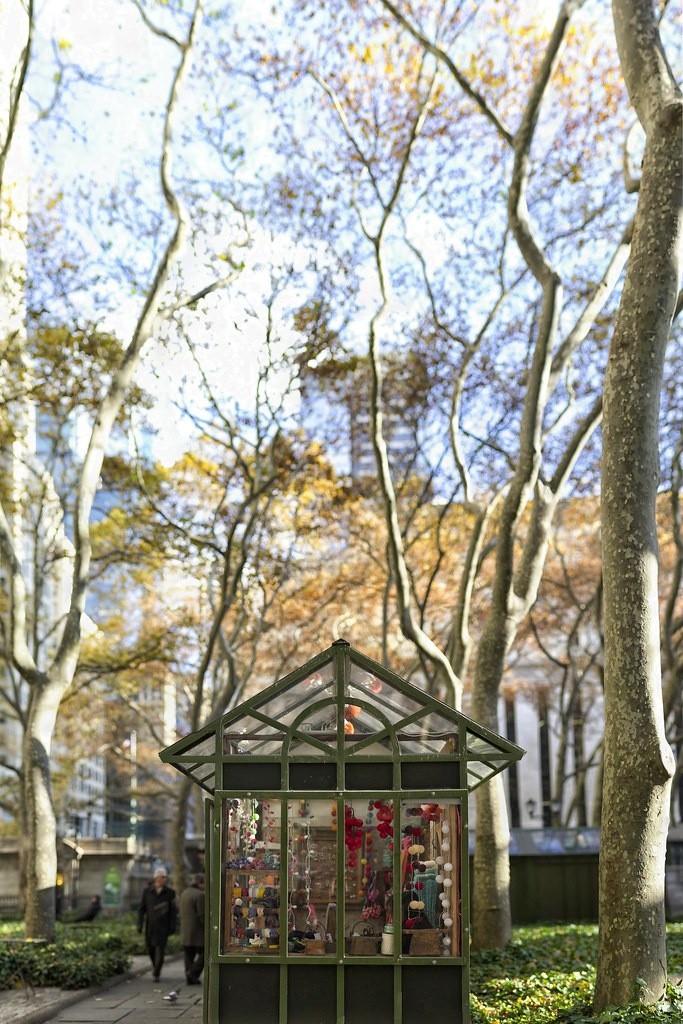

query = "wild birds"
(162, 988), (181, 1005)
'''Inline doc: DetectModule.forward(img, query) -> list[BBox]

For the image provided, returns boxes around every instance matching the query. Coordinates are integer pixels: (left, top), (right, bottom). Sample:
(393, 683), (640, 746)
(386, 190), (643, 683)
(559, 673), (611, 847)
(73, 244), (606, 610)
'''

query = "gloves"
(170, 928), (176, 935)
(137, 925), (143, 934)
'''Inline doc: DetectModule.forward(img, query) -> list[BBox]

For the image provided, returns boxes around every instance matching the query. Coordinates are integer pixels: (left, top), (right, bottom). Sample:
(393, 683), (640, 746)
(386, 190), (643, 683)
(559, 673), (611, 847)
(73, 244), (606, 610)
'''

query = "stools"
(0, 937), (48, 1000)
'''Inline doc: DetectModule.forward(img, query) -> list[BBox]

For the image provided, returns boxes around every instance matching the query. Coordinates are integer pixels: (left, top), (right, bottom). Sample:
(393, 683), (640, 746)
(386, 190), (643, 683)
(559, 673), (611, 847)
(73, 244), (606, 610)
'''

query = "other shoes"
(186, 971), (202, 985)
(153, 976), (160, 983)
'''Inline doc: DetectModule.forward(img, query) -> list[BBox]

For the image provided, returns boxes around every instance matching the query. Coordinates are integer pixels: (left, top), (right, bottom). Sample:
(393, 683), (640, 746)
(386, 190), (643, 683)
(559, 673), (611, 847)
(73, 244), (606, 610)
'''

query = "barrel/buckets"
(302, 921), (327, 955)
(350, 921), (378, 956)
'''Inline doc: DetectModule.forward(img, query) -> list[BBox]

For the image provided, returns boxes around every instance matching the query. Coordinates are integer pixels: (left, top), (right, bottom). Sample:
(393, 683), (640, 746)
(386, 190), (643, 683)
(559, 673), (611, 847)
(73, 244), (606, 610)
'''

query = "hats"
(153, 867), (168, 879)
(189, 874), (204, 886)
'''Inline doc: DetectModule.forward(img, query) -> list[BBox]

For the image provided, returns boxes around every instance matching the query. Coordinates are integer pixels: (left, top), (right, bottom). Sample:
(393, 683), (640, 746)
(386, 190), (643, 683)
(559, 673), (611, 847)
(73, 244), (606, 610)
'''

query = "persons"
(55, 885), (101, 922)
(136, 867), (181, 983)
(385, 891), (432, 955)
(175, 875), (205, 986)
